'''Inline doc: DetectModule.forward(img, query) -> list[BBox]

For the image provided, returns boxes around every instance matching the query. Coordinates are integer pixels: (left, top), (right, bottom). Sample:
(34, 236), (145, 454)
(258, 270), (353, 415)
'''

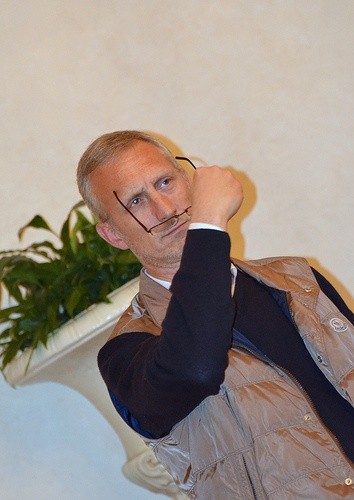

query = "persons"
(76, 130), (354, 499)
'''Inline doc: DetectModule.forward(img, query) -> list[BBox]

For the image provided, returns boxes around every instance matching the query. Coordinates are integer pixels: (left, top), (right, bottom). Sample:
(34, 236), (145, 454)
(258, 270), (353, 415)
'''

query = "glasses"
(114, 156), (198, 238)
